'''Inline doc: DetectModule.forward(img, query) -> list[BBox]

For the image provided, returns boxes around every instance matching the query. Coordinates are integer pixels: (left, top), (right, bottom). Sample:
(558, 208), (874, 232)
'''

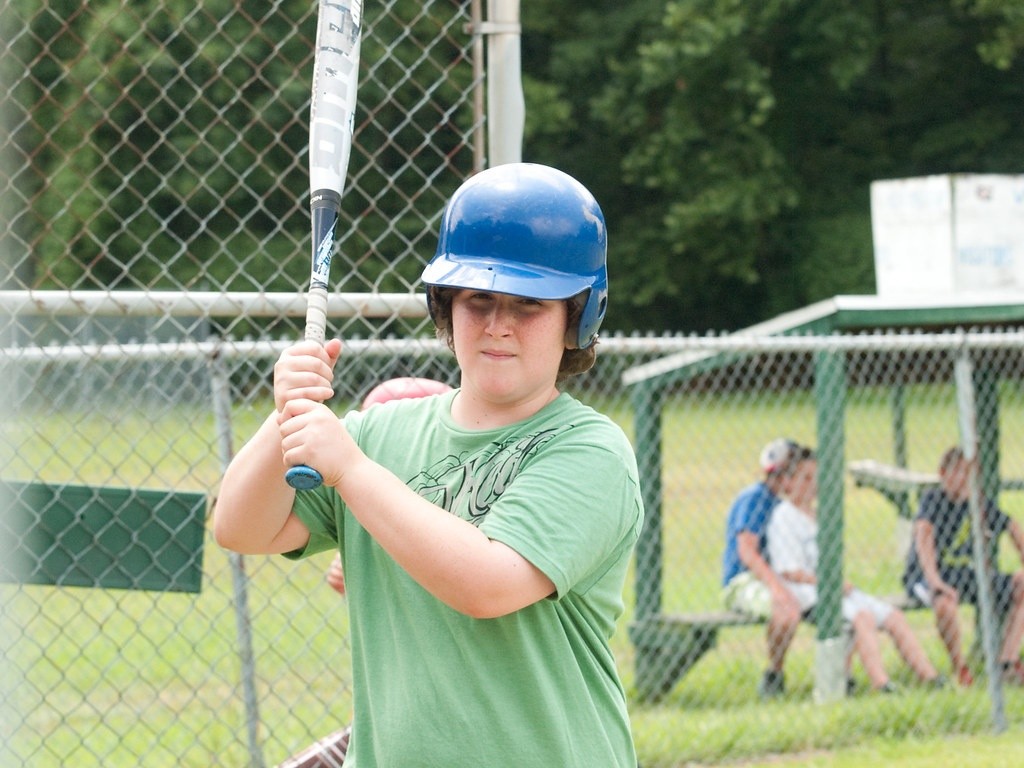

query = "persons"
(723, 440), (858, 699)
(900, 449), (1023, 690)
(213, 162), (644, 768)
(768, 447), (956, 697)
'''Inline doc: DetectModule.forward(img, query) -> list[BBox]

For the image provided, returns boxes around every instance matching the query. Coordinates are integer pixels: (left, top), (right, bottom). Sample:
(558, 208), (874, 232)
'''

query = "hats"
(761, 439), (809, 475)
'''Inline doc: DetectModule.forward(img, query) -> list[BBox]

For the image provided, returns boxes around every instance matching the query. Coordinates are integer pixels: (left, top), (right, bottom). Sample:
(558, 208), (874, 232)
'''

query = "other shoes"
(876, 681), (898, 696)
(918, 672), (948, 692)
(760, 668), (785, 700)
(846, 677), (857, 696)
(955, 666), (973, 689)
(998, 661), (1024, 684)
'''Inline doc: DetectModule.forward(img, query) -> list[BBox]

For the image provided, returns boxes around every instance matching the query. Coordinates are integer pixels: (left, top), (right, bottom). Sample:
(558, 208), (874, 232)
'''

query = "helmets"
(420, 163), (608, 351)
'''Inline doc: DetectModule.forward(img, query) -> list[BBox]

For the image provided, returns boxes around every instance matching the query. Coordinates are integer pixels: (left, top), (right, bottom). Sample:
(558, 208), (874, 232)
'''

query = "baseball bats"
(284, 0), (363, 488)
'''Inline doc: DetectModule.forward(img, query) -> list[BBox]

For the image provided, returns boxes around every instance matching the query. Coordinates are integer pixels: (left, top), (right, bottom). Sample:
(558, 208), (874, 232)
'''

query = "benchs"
(626, 593), (1004, 710)
